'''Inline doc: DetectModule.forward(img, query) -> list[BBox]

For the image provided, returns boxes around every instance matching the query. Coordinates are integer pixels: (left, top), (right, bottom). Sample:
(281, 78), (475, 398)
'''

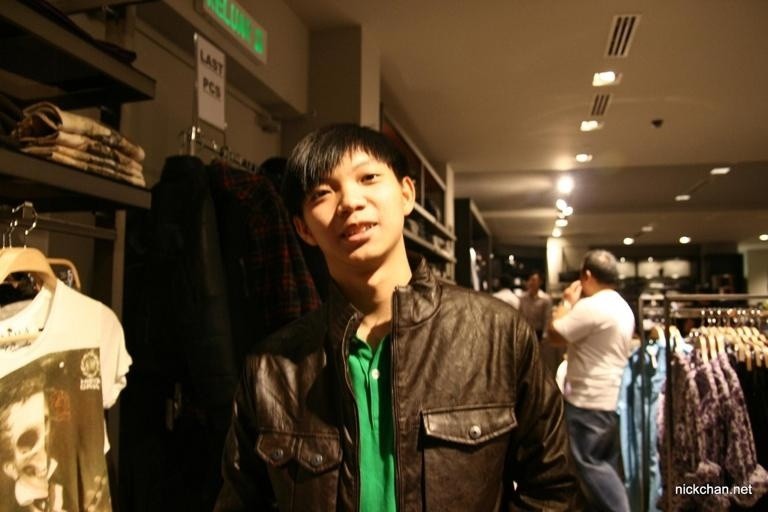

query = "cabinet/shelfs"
(0, 0), (158, 512)
(382, 110), (457, 287)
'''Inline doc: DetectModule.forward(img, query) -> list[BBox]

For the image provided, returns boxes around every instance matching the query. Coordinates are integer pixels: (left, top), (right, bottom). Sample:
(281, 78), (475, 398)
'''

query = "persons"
(519, 270), (551, 345)
(214, 124), (582, 512)
(547, 249), (635, 512)
(491, 275), (520, 309)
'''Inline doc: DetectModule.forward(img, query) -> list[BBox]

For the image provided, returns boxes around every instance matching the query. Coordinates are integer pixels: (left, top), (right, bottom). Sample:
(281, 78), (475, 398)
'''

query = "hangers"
(686, 308), (768, 371)
(0, 204), (81, 291)
(179, 126), (259, 177)
